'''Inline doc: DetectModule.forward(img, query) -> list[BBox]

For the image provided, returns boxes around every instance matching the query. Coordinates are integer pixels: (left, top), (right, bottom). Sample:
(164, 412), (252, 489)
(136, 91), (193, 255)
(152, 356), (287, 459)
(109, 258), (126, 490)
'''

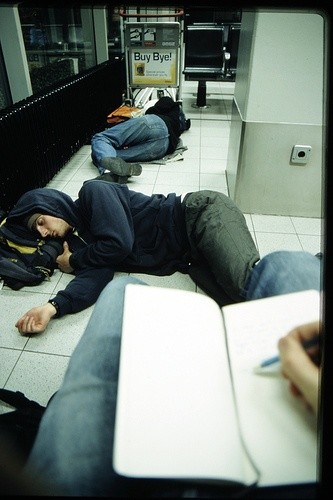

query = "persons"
(0, 180), (323, 336)
(90, 96), (191, 184)
(20, 250), (324, 494)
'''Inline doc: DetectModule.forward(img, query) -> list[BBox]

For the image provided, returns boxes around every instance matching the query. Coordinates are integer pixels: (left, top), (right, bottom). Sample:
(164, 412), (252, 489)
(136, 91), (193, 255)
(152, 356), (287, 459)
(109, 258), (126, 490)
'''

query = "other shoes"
(84, 172), (130, 184)
(101, 156), (143, 176)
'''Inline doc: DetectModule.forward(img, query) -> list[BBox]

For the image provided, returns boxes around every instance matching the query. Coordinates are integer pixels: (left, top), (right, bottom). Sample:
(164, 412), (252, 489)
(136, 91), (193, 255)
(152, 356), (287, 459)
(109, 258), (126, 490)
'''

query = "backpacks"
(0, 216), (56, 291)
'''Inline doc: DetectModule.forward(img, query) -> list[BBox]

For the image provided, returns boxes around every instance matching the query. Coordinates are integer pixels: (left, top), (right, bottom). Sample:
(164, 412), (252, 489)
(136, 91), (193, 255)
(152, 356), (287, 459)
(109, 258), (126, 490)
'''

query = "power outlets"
(291, 145), (311, 164)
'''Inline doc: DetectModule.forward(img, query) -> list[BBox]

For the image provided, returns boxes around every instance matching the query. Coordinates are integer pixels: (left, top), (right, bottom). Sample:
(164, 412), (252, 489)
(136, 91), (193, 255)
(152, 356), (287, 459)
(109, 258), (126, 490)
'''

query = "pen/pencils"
(257, 342), (314, 370)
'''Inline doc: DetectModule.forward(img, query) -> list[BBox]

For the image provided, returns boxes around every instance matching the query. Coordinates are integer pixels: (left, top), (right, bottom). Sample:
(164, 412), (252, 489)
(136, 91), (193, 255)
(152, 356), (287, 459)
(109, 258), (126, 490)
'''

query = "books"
(112, 283), (322, 488)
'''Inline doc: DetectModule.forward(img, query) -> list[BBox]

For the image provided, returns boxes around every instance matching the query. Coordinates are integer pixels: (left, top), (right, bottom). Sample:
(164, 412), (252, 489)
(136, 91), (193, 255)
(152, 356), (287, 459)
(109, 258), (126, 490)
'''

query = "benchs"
(181, 21), (241, 109)
